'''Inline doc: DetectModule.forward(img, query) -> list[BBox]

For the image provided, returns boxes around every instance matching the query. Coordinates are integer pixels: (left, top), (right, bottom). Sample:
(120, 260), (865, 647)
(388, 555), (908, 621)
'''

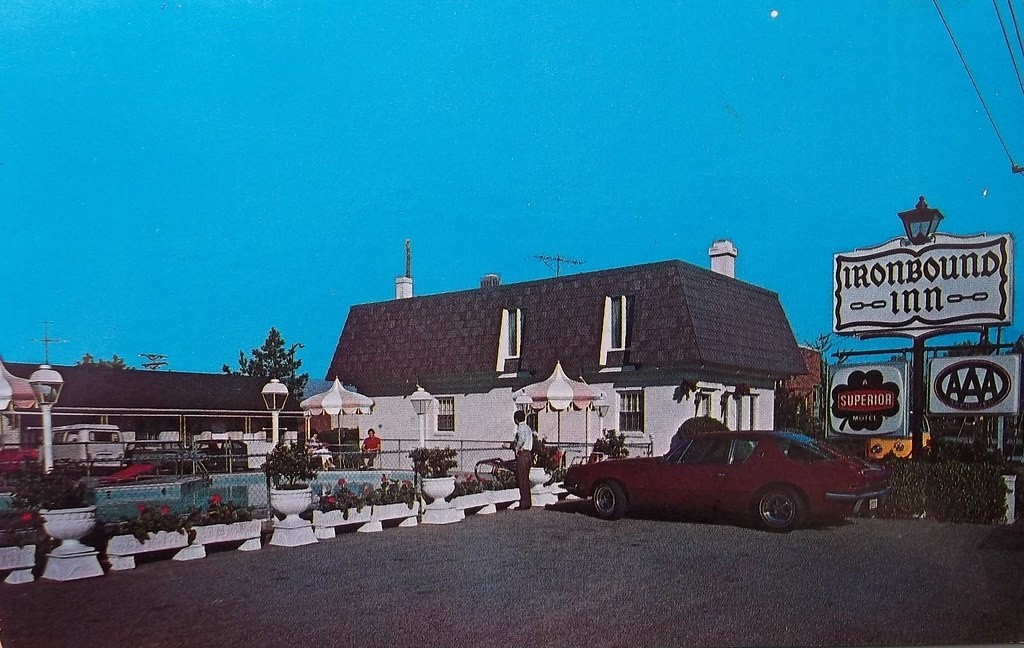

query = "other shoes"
(360, 465), (366, 468)
(514, 506), (530, 511)
(367, 466), (373, 469)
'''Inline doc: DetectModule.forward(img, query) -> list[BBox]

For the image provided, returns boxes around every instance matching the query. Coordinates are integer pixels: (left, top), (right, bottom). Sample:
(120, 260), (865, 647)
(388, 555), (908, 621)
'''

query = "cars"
(565, 426), (896, 532)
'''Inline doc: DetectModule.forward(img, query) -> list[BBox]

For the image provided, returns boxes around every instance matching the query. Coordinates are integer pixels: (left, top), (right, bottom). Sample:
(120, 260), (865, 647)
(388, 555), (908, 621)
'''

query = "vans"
(37, 422), (128, 476)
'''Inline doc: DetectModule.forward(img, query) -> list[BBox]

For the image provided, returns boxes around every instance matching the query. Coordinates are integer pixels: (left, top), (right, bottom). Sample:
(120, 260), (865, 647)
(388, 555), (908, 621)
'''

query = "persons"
(510, 410), (532, 510)
(304, 428), (335, 471)
(358, 428), (380, 470)
